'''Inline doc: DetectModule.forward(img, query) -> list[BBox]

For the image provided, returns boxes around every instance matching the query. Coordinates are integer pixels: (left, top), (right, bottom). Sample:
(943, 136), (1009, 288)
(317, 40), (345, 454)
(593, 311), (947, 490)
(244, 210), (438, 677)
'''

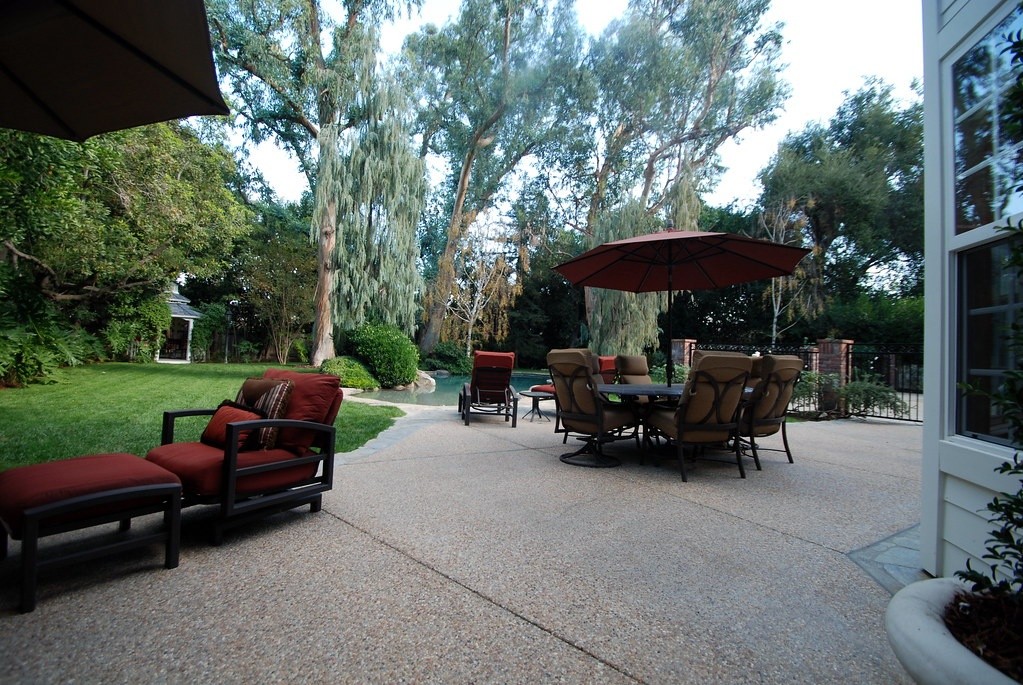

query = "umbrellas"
(0, 0), (231, 145)
(551, 220), (812, 388)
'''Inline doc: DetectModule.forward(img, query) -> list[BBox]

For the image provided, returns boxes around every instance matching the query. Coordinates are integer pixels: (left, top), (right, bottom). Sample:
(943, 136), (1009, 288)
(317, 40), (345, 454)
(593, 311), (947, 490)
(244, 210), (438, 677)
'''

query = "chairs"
(614, 355), (652, 403)
(641, 350), (751, 483)
(692, 354), (804, 470)
(144, 367), (343, 546)
(546, 348), (634, 468)
(458, 350), (518, 427)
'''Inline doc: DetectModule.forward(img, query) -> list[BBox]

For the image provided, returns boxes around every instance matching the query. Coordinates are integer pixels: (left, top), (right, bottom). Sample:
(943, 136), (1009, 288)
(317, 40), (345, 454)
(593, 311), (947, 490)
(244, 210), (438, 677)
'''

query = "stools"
(0, 451), (182, 612)
(530, 384), (557, 413)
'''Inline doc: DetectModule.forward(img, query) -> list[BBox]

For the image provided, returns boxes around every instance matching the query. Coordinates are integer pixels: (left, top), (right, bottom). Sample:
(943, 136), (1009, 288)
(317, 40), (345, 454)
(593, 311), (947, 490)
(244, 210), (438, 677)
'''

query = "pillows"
(234, 376), (294, 446)
(200, 399), (269, 452)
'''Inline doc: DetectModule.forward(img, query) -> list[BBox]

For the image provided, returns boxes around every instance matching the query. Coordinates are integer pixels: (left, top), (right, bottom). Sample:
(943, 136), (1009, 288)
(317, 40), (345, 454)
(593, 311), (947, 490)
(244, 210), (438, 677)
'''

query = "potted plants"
(884, 217), (1023, 685)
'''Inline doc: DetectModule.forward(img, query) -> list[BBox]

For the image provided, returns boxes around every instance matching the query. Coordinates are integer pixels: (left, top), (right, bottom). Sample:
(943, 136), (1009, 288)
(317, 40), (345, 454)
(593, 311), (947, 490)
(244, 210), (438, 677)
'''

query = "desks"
(519, 392), (554, 422)
(593, 384), (754, 466)
